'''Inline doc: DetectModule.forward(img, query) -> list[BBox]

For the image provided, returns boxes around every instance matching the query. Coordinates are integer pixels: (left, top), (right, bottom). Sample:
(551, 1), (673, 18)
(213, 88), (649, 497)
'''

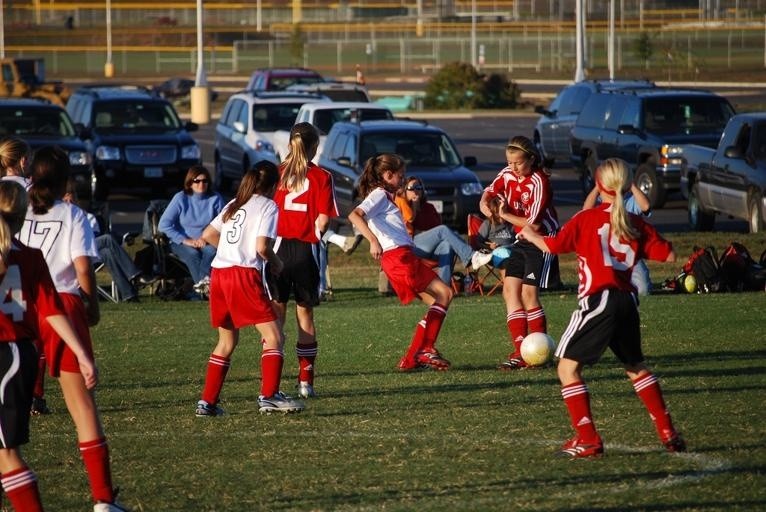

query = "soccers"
(520, 332), (555, 367)
(685, 275), (698, 293)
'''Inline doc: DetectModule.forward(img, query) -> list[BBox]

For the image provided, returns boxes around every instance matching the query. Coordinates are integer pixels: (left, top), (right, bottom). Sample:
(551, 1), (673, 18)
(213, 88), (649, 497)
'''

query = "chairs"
(77, 201), (120, 304)
(467, 214), (503, 296)
(413, 199), (461, 296)
(141, 200), (191, 297)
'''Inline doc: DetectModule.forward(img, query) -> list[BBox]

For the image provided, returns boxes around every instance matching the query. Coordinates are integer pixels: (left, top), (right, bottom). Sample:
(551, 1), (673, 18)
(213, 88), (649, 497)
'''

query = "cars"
(153, 78), (216, 102)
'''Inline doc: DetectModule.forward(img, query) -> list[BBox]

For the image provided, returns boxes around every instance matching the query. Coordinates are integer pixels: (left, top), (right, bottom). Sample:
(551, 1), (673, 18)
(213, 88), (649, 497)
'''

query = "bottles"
(463, 273), (472, 297)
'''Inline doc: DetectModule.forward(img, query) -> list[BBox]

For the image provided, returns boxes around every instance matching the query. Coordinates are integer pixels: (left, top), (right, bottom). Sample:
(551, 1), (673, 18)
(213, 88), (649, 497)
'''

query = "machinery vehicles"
(0, 56), (68, 107)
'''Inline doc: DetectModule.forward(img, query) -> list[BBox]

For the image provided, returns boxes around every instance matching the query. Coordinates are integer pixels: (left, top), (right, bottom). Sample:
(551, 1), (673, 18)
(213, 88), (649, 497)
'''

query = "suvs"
(532, 78), (659, 169)
(0, 96), (92, 208)
(68, 84), (204, 204)
(247, 65), (323, 95)
(571, 90), (739, 209)
(212, 90), (331, 200)
(316, 111), (484, 234)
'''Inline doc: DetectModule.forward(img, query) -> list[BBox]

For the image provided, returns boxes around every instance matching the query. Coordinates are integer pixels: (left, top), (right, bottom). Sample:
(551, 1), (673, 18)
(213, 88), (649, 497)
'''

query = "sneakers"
(550, 433), (603, 459)
(196, 401), (226, 417)
(258, 380), (315, 413)
(398, 355), (427, 373)
(496, 350), (525, 371)
(663, 435), (684, 451)
(31, 398), (56, 415)
(472, 250), (492, 270)
(193, 276), (209, 294)
(92, 487), (129, 512)
(414, 350), (450, 371)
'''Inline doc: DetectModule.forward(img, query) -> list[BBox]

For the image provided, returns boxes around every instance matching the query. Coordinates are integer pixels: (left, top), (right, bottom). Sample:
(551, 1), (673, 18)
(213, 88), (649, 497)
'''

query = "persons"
(583, 182), (653, 296)
(515, 155), (685, 460)
(347, 152), (563, 370)
(159, 166), (227, 299)
(263, 119), (341, 402)
(1, 135), (159, 412)
(13, 143), (135, 511)
(193, 160), (304, 419)
(0, 180), (99, 512)
(312, 228), (364, 298)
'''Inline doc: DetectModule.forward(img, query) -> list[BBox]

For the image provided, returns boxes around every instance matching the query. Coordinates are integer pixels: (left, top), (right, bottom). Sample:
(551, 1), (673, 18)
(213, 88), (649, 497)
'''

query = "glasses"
(193, 179), (208, 183)
(406, 186), (421, 190)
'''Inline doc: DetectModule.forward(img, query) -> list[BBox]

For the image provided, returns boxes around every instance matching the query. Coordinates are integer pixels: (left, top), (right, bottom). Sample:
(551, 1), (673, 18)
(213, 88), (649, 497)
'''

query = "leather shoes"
(137, 276), (159, 285)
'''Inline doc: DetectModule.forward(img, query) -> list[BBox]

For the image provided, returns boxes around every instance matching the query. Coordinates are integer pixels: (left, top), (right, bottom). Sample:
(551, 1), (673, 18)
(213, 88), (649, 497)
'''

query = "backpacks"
(720, 242), (766, 292)
(683, 246), (723, 293)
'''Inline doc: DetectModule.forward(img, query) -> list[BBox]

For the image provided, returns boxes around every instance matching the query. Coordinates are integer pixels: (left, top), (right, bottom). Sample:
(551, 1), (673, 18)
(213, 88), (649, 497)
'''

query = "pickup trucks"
(679, 114), (766, 239)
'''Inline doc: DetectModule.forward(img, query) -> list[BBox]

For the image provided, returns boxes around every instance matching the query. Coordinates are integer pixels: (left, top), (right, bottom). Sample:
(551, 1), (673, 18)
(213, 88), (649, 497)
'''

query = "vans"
(294, 104), (394, 167)
(285, 81), (370, 103)
